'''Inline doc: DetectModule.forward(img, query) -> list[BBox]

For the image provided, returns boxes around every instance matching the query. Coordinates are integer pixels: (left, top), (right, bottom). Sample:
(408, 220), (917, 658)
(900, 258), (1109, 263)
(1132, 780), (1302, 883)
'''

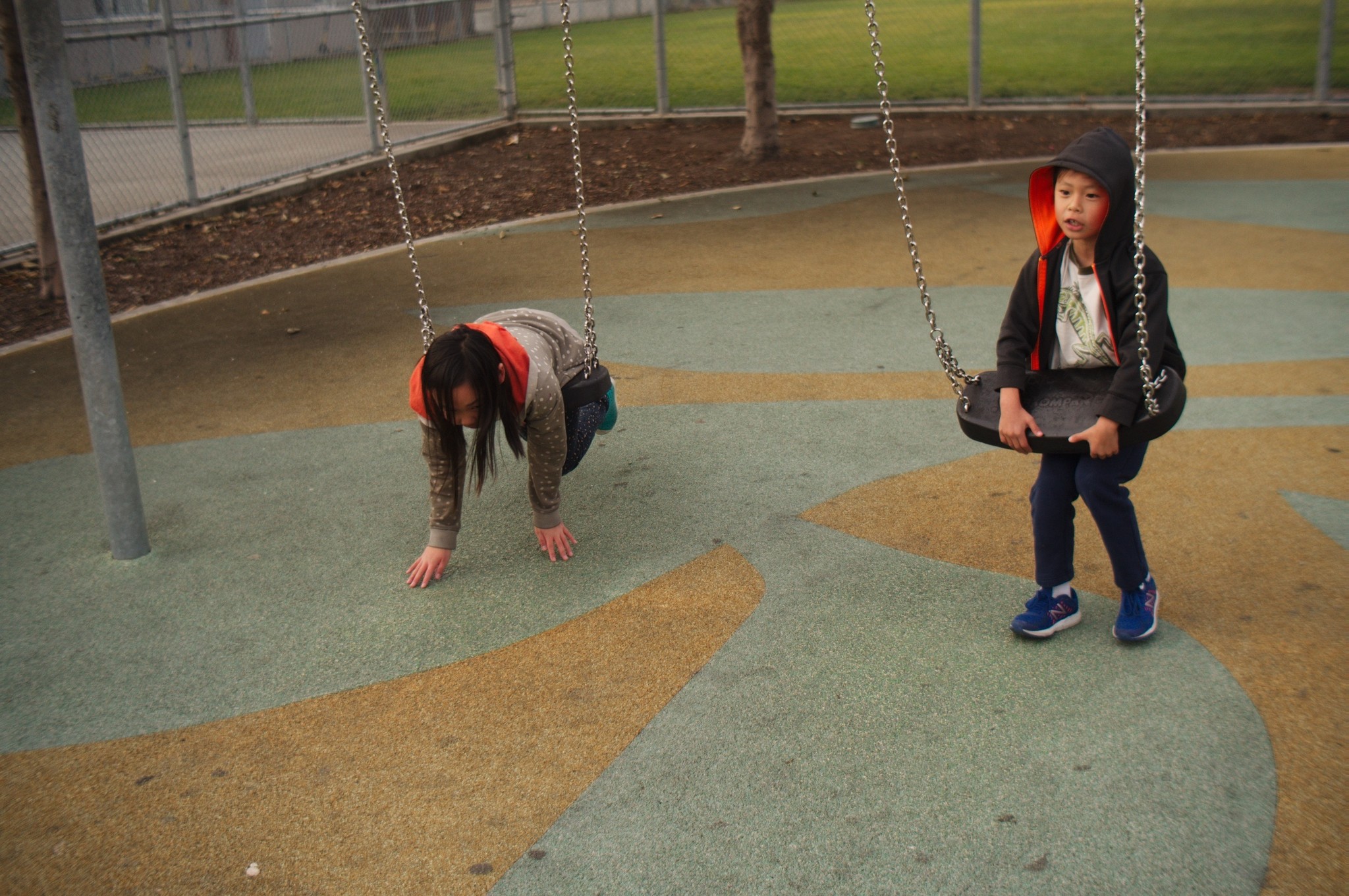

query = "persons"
(995, 130), (1188, 641)
(405, 307), (619, 589)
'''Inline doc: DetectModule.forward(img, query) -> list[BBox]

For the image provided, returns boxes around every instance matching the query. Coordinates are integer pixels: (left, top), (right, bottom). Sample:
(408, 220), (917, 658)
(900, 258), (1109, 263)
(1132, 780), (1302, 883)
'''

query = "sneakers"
(1114, 576), (1159, 640)
(1010, 587), (1080, 637)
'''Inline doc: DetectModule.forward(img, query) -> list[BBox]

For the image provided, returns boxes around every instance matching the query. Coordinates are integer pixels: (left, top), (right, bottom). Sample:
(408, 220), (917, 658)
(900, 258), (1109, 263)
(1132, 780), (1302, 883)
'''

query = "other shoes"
(595, 376), (617, 435)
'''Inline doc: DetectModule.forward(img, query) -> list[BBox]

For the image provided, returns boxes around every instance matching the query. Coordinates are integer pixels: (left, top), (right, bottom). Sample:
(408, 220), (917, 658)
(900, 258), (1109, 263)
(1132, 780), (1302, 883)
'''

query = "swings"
(860, 1), (1189, 459)
(346, 0), (617, 421)
